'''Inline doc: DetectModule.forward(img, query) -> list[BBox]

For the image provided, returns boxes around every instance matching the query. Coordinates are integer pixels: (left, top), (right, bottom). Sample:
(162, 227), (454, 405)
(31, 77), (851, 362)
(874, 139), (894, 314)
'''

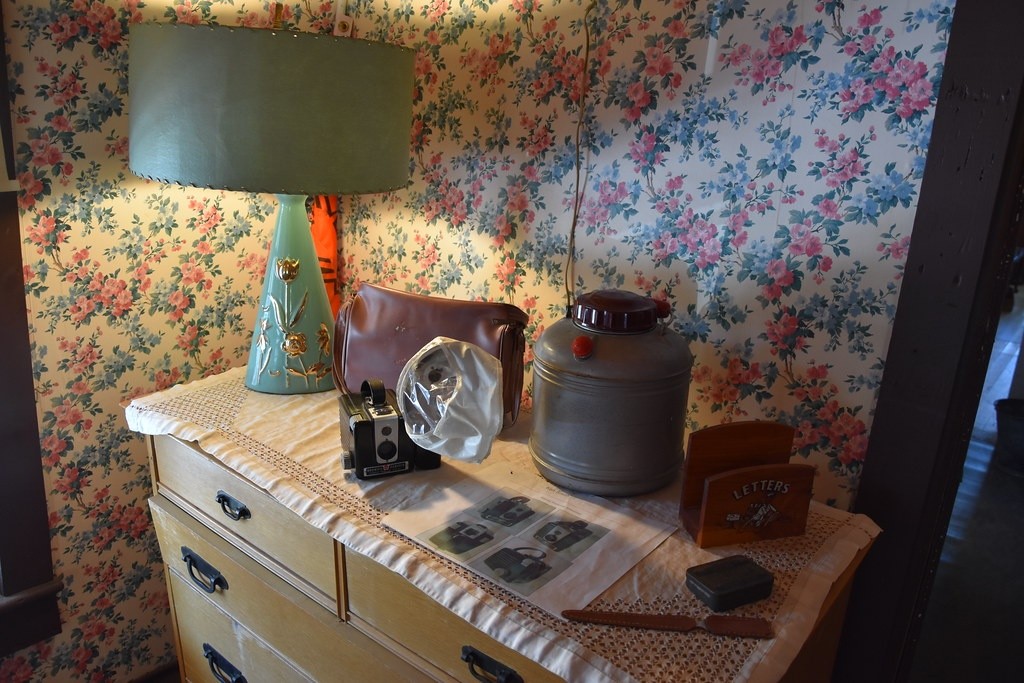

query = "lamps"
(128, 23), (416, 395)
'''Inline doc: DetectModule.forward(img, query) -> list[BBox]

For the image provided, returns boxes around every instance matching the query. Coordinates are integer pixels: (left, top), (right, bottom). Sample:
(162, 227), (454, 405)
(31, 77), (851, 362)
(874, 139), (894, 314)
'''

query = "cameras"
(428, 497), (593, 584)
(338, 379), (441, 483)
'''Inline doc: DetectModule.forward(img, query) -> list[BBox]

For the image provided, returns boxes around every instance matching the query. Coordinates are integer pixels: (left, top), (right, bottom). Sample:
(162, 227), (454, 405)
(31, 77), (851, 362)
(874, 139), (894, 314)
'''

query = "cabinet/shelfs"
(126, 367), (884, 682)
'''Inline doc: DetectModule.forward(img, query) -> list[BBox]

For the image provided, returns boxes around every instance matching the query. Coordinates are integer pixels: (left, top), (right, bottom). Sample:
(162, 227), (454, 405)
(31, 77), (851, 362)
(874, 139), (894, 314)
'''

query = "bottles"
(527, 289), (693, 496)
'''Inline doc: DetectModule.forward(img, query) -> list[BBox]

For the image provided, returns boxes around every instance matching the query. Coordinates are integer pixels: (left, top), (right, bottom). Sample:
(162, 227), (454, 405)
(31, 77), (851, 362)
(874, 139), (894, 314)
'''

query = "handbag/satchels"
(332, 281), (528, 427)
